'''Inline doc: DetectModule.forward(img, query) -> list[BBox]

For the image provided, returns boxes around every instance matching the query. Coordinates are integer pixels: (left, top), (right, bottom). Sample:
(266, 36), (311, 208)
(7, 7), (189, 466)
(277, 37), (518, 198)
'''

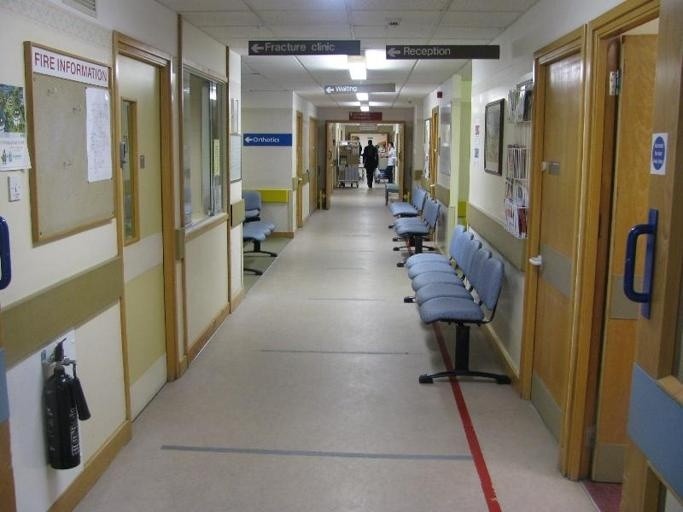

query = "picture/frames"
(483, 97), (505, 176)
(22, 36), (120, 248)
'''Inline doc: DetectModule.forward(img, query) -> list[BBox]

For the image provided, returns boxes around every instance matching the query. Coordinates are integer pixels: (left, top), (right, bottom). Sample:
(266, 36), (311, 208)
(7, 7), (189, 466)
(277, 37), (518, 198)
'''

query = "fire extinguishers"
(43, 337), (91, 470)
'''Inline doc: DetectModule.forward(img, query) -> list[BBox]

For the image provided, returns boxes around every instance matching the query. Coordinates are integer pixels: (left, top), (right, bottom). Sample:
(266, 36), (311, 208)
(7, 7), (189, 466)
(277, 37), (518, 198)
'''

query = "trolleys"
(373, 166), (393, 183)
(336, 152), (365, 188)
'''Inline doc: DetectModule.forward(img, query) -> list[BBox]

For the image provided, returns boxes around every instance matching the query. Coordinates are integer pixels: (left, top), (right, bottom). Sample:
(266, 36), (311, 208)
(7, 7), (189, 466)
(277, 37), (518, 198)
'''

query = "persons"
(362, 139), (378, 191)
(354, 136), (362, 157)
(379, 141), (395, 181)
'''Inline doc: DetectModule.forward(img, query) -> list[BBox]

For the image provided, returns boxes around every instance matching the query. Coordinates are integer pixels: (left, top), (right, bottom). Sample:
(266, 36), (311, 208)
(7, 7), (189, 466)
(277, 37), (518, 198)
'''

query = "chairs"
(385, 182), (511, 384)
(242, 190), (277, 275)
